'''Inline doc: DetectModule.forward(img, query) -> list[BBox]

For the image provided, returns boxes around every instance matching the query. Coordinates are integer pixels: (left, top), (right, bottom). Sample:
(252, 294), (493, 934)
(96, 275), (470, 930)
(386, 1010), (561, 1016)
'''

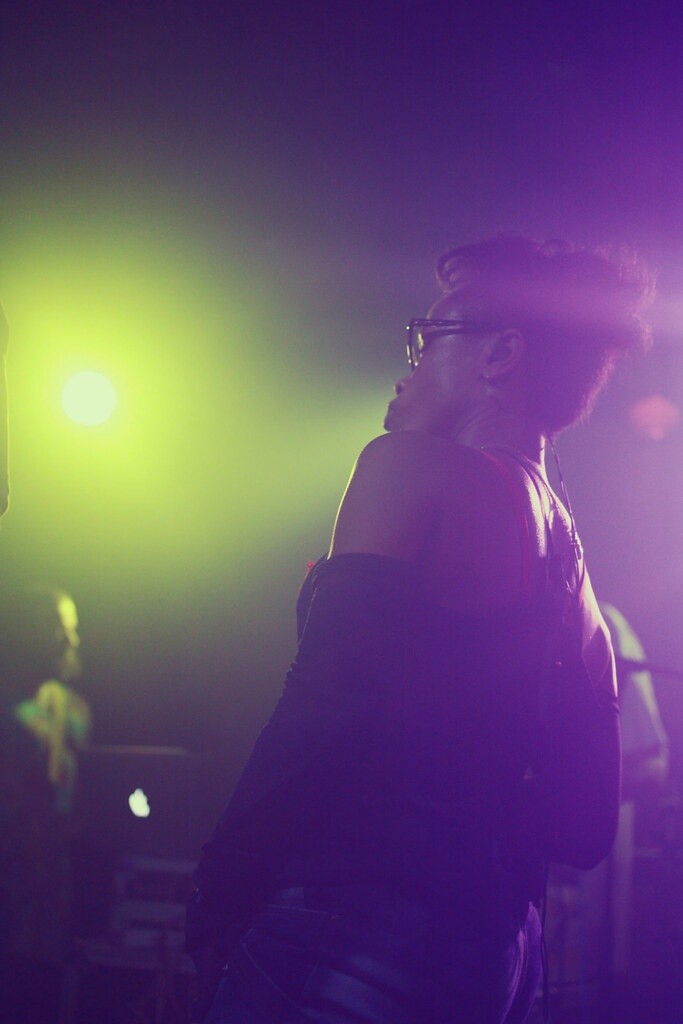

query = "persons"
(535, 603), (668, 1024)
(12, 583), (91, 811)
(188, 238), (651, 1024)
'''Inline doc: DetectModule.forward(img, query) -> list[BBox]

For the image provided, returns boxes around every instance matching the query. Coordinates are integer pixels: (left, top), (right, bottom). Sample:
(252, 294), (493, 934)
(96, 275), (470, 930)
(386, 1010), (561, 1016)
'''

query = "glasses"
(405, 317), (533, 370)
